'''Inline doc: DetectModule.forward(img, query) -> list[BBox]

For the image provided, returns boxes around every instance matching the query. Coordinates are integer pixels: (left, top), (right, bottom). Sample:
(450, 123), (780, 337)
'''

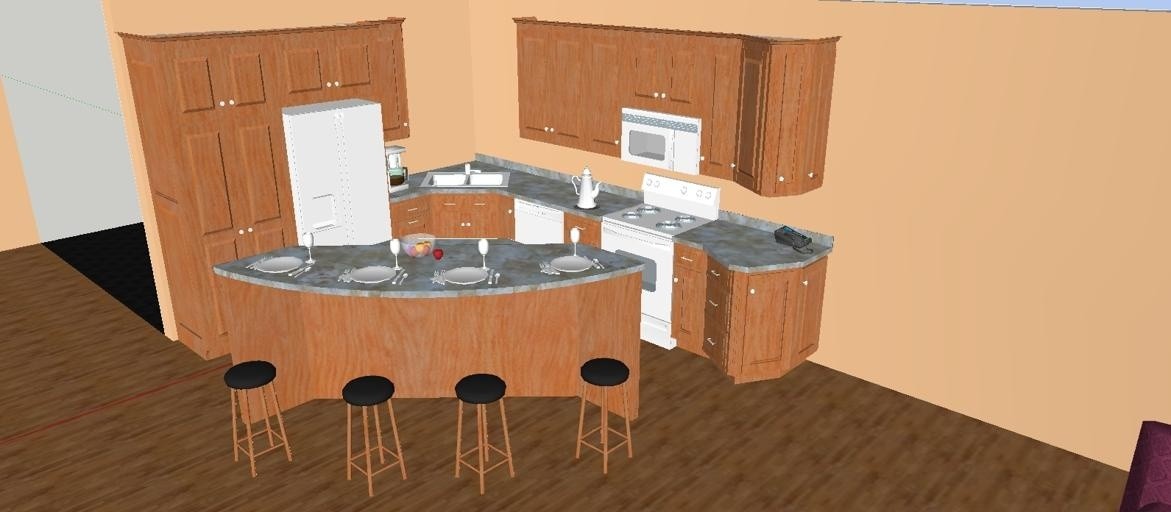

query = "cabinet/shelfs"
(511, 15), (842, 198)
(114, 18), (411, 363)
(670, 243), (829, 385)
(563, 212), (601, 249)
(390, 195), (515, 240)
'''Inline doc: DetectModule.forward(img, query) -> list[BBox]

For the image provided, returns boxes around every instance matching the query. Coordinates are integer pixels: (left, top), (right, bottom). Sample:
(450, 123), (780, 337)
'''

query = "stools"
(342, 375), (407, 499)
(454, 374), (515, 497)
(575, 358), (633, 474)
(223, 360), (293, 478)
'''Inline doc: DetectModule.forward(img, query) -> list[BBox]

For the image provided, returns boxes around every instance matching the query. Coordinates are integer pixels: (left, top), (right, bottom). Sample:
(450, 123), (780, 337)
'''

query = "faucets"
(465, 163), (472, 176)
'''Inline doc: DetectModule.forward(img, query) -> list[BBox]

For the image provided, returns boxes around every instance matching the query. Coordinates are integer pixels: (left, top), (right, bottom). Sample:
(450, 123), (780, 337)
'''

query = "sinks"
(419, 171), (466, 188)
(466, 172), (512, 188)
